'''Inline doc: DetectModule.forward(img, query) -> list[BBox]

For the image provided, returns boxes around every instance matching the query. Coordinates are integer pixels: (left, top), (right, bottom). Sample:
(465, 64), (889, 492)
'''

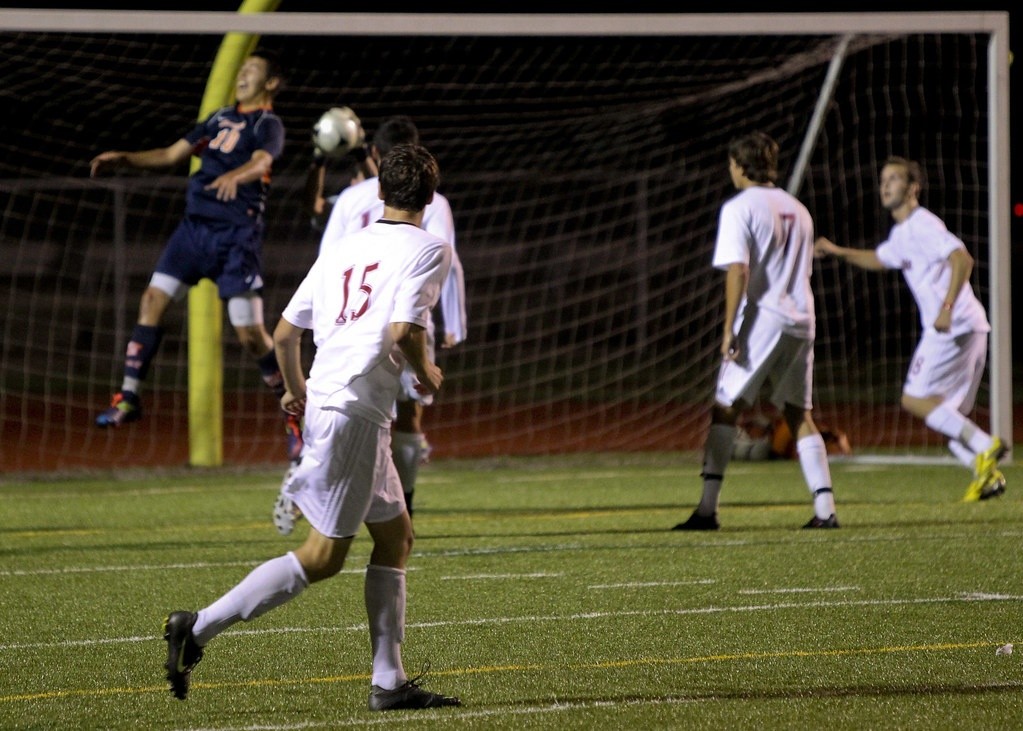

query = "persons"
(88, 48), (302, 459)
(814, 157), (1011, 502)
(670, 134), (841, 532)
(163, 118), (467, 712)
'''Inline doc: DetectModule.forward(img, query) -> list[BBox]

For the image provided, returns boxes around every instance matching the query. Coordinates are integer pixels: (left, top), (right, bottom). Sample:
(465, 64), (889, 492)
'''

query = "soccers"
(311, 106), (364, 164)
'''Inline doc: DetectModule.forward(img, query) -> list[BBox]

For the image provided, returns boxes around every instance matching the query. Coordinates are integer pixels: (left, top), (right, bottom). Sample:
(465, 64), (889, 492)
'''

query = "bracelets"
(943, 301), (951, 310)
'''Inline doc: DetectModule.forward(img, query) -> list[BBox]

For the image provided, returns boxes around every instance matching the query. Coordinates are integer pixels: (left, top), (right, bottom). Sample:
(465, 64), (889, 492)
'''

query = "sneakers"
(162, 611), (207, 700)
(368, 660), (462, 712)
(284, 415), (303, 462)
(802, 513), (838, 528)
(94, 389), (143, 430)
(965, 470), (1006, 502)
(672, 508), (720, 530)
(972, 435), (1008, 491)
